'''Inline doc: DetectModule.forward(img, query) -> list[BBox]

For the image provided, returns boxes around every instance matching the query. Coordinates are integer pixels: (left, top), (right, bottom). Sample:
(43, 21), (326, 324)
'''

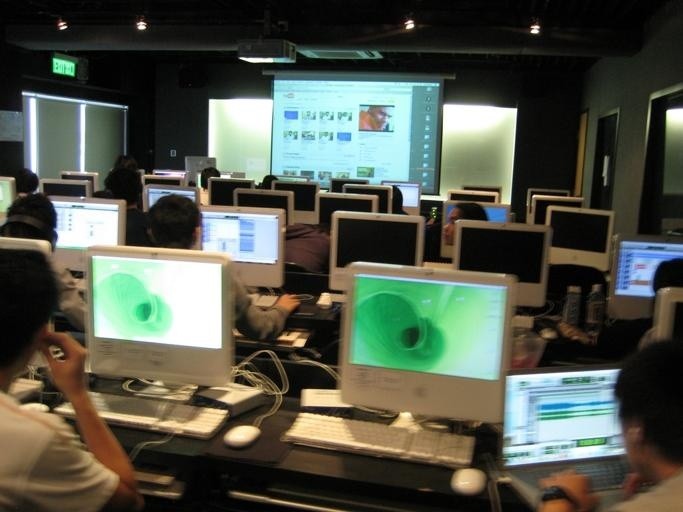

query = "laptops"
(185, 157), (216, 173)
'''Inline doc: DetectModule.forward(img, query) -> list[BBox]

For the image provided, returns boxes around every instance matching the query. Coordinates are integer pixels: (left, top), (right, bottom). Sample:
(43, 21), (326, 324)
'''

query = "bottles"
(563, 286), (582, 325)
(585, 284), (605, 334)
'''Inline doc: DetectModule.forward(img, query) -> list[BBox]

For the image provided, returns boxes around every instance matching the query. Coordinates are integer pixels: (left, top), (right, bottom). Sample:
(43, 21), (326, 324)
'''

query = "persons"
(0, 249), (146, 507)
(11, 170), (39, 199)
(112, 155), (136, 170)
(538, 336), (683, 512)
(144, 197), (300, 340)
(442, 202), (489, 247)
(359, 105), (395, 131)
(93, 166), (151, 245)
(392, 185), (435, 226)
(554, 258), (682, 345)
(199, 166), (221, 204)
(1, 196), (84, 334)
(283, 213), (330, 273)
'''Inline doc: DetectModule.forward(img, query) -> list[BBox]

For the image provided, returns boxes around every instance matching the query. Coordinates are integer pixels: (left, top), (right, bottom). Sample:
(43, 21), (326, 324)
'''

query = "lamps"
(135, 15), (148, 31)
(529, 19), (541, 34)
(56, 18), (68, 30)
(404, 15), (416, 30)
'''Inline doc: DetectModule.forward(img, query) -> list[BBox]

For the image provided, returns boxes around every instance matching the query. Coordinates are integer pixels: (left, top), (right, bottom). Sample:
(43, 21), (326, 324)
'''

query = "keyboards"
(527, 460), (656, 493)
(284, 412), (476, 468)
(62, 391), (231, 442)
(73, 277), (86, 294)
(513, 315), (536, 331)
(246, 293), (279, 310)
(7, 378), (45, 401)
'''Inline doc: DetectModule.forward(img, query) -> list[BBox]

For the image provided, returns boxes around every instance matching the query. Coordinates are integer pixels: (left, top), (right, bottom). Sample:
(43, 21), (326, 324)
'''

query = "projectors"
(236, 38), (297, 64)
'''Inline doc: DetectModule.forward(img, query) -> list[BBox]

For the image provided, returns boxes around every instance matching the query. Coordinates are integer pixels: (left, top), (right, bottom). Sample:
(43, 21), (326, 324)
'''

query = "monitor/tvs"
(543, 205), (613, 272)
(498, 361), (628, 470)
(207, 177), (255, 206)
(328, 210), (426, 292)
(194, 204), (285, 290)
(440, 200), (511, 259)
(86, 245), (235, 402)
(141, 170), (201, 217)
(654, 287), (683, 342)
(47, 195), (127, 273)
(329, 178), (369, 193)
(59, 171), (100, 193)
(233, 188), (295, 226)
(526, 187), (585, 228)
(342, 183), (393, 214)
(0, 236), (53, 257)
(452, 220), (552, 307)
(39, 178), (93, 198)
(382, 181), (421, 215)
(0, 176), (18, 226)
(607, 234), (682, 320)
(340, 261), (517, 426)
(448, 185), (502, 203)
(271, 180), (321, 225)
(314, 190), (379, 235)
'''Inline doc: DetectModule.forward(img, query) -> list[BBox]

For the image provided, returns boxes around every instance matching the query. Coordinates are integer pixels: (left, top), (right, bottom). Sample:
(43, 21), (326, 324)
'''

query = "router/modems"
(299, 388), (354, 418)
(194, 382), (266, 418)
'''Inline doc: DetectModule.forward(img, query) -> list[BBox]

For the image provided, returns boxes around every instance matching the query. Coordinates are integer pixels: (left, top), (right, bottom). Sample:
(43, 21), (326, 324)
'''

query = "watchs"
(537, 485), (583, 508)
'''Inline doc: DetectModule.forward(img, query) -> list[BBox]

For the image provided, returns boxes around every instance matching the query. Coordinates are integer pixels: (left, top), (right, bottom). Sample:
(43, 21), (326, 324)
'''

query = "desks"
(0, 367), (510, 511)
(0, 268), (629, 392)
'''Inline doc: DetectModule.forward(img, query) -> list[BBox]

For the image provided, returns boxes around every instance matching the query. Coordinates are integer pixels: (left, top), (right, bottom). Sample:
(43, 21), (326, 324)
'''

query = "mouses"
(20, 402), (51, 413)
(450, 468), (488, 496)
(541, 328), (558, 340)
(223, 425), (261, 448)
